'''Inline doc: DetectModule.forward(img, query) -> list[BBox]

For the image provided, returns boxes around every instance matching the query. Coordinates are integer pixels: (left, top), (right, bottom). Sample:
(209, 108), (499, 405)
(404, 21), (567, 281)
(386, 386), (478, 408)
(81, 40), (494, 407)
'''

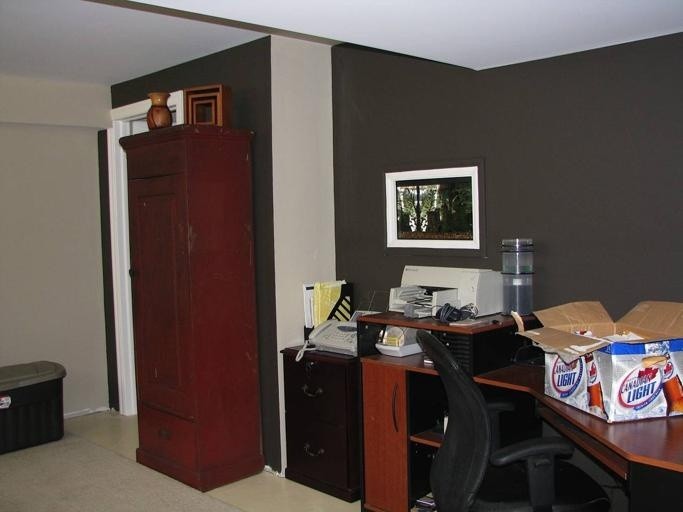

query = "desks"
(356, 311), (683, 512)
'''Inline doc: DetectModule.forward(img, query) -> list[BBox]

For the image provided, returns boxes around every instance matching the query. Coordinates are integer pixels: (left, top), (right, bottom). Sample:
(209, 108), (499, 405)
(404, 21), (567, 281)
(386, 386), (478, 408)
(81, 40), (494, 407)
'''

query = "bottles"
(583, 350), (609, 420)
(654, 353), (683, 416)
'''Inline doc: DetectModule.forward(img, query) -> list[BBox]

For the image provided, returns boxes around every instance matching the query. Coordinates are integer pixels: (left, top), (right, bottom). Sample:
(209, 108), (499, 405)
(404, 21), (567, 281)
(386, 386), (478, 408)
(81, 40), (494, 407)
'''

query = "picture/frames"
(382, 165), (482, 250)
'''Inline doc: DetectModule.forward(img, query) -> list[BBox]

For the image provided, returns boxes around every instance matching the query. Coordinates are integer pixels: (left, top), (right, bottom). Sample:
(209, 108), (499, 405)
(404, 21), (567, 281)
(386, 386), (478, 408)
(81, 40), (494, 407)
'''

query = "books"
(303, 280), (350, 329)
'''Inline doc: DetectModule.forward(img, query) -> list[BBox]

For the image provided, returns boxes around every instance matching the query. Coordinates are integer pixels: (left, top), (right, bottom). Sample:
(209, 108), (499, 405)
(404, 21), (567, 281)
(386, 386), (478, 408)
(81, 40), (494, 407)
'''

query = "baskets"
(304, 282), (354, 339)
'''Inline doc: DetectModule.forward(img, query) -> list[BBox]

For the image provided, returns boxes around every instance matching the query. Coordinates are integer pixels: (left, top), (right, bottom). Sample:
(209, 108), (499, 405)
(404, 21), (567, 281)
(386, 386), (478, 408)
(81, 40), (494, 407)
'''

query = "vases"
(143, 92), (171, 131)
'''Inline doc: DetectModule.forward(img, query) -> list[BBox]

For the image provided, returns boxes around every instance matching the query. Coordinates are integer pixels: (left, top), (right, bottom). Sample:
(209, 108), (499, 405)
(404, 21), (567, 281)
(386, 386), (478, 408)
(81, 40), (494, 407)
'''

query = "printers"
(388, 265), (503, 319)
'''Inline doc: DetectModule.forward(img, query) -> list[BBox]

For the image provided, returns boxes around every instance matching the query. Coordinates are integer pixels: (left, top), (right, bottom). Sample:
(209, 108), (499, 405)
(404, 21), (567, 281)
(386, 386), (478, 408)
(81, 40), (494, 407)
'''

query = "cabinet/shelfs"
(118, 125), (264, 493)
(280, 344), (363, 503)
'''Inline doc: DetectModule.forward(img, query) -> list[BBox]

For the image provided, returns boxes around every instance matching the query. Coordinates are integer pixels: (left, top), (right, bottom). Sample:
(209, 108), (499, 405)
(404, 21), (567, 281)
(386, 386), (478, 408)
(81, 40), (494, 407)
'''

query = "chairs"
(402, 330), (575, 512)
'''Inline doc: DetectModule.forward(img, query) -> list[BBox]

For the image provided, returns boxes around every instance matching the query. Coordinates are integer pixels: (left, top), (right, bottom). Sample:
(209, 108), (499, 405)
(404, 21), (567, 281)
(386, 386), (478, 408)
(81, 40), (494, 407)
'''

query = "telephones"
(308, 319), (359, 356)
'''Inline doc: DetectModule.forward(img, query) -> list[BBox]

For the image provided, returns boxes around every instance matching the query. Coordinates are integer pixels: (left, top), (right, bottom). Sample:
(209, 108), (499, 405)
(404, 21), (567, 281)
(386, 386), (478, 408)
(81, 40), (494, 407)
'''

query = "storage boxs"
(0, 360), (67, 455)
(514, 299), (683, 423)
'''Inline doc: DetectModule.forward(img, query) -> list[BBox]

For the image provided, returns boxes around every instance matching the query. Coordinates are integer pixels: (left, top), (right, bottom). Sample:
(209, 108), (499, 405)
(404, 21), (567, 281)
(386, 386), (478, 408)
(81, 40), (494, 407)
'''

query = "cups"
(500, 239), (535, 316)
